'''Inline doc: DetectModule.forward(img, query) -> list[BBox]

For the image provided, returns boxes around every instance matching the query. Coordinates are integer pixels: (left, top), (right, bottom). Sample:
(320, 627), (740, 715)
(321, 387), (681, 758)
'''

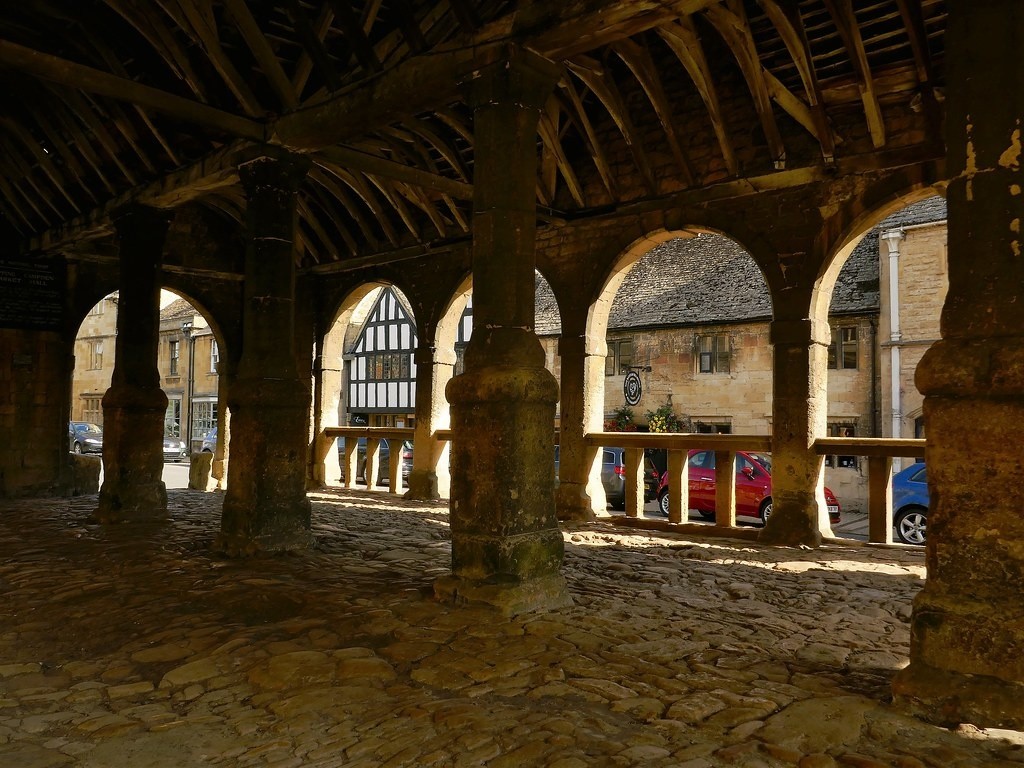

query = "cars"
(68, 420), (103, 455)
(554, 442), (660, 511)
(891, 462), (928, 547)
(336, 436), (413, 486)
(163, 427), (187, 463)
(656, 447), (841, 528)
(201, 426), (218, 454)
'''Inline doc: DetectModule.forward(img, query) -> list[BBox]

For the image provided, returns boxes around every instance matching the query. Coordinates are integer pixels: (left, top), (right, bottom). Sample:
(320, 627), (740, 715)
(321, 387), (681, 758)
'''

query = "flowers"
(604, 402), (639, 432)
(643, 400), (686, 433)
